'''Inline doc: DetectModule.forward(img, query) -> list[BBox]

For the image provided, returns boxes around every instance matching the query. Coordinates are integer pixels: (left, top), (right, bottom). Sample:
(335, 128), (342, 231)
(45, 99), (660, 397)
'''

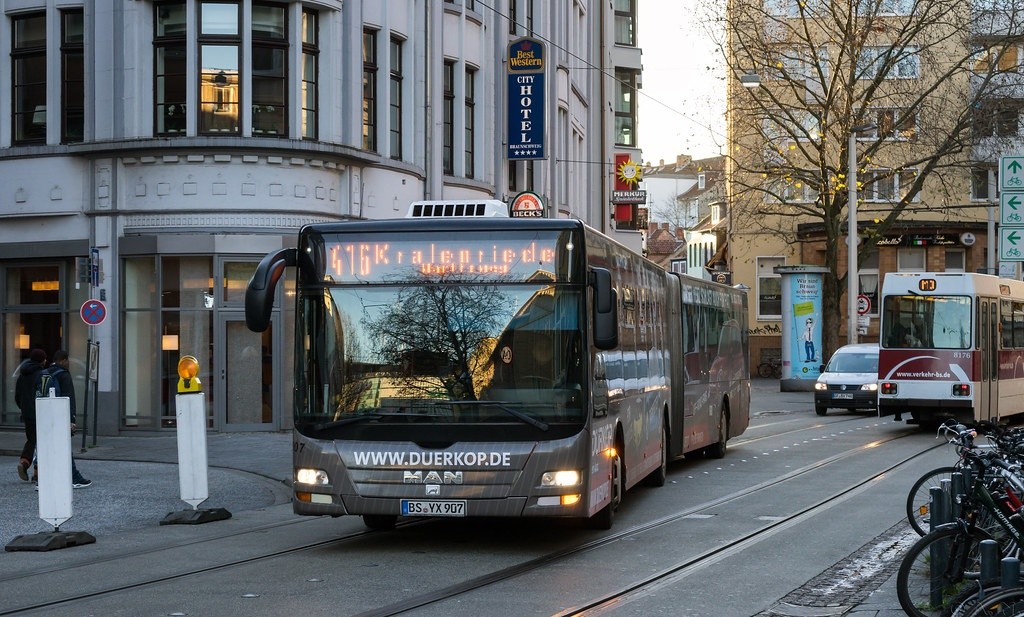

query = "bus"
(878, 271), (1024, 430)
(243, 199), (752, 539)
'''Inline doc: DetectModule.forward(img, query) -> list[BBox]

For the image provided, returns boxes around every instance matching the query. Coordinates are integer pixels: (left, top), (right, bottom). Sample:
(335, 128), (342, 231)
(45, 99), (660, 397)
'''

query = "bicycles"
(757, 358), (782, 379)
(894, 420), (1023, 617)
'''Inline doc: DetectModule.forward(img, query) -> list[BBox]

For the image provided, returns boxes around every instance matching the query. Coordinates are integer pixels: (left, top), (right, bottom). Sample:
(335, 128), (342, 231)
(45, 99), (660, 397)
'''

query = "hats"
(30, 349), (47, 364)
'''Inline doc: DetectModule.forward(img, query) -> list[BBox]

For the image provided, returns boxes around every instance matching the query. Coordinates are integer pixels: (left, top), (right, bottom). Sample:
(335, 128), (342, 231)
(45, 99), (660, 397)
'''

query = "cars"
(813, 343), (882, 416)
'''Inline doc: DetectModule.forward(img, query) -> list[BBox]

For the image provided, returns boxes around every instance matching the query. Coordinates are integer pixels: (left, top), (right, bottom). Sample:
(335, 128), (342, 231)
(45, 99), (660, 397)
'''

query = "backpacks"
(35, 369), (67, 398)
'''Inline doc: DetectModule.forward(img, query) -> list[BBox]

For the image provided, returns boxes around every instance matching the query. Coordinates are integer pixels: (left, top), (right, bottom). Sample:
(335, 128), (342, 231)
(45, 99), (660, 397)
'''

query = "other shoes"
(31, 465), (38, 482)
(18, 459), (30, 481)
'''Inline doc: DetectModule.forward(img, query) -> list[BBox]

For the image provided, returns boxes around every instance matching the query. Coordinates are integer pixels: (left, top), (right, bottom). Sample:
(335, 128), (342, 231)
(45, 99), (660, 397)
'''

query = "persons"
(42, 349), (92, 489)
(888, 323), (911, 347)
(15, 349), (48, 493)
(527, 333), (582, 385)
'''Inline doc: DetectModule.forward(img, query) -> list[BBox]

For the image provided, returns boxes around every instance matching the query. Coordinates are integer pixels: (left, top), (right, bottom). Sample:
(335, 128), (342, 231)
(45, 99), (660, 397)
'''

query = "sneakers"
(35, 482), (38, 491)
(72, 472), (92, 488)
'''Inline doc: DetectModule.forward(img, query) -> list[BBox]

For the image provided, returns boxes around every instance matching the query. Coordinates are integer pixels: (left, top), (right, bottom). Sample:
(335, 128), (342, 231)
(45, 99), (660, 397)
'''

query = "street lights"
(847, 121), (878, 349)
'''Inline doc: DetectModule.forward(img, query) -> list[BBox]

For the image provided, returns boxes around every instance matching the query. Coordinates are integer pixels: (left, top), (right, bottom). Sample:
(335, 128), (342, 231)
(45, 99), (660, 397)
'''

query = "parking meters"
(155, 355), (232, 525)
